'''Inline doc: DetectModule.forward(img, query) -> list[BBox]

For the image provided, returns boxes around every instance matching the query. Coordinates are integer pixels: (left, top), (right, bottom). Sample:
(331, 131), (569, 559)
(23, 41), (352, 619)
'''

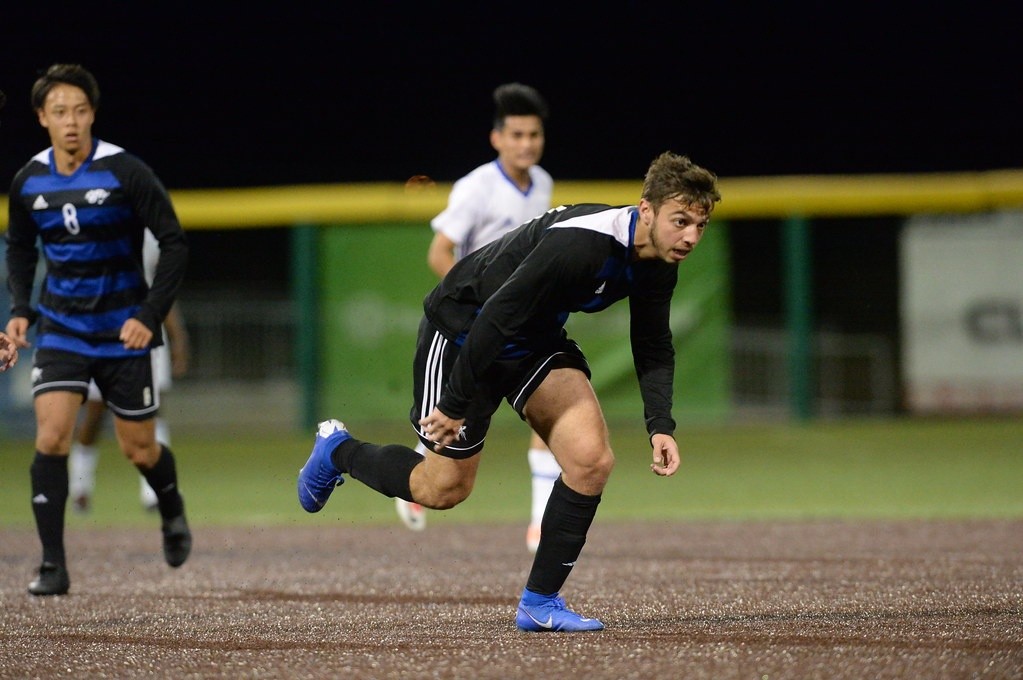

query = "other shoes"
(526, 526), (540, 553)
(397, 496), (427, 532)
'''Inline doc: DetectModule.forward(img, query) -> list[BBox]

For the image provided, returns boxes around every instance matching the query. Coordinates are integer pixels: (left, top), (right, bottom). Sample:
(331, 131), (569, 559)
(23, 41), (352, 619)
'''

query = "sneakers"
(298, 418), (353, 513)
(161, 505), (193, 568)
(515, 588), (605, 633)
(28, 568), (69, 595)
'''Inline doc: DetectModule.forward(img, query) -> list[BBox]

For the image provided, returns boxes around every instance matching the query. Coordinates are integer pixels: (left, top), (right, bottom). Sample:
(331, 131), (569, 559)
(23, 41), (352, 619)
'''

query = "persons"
(0, 331), (18, 372)
(6, 65), (192, 596)
(298, 154), (721, 632)
(69, 229), (185, 510)
(396, 84), (562, 551)
